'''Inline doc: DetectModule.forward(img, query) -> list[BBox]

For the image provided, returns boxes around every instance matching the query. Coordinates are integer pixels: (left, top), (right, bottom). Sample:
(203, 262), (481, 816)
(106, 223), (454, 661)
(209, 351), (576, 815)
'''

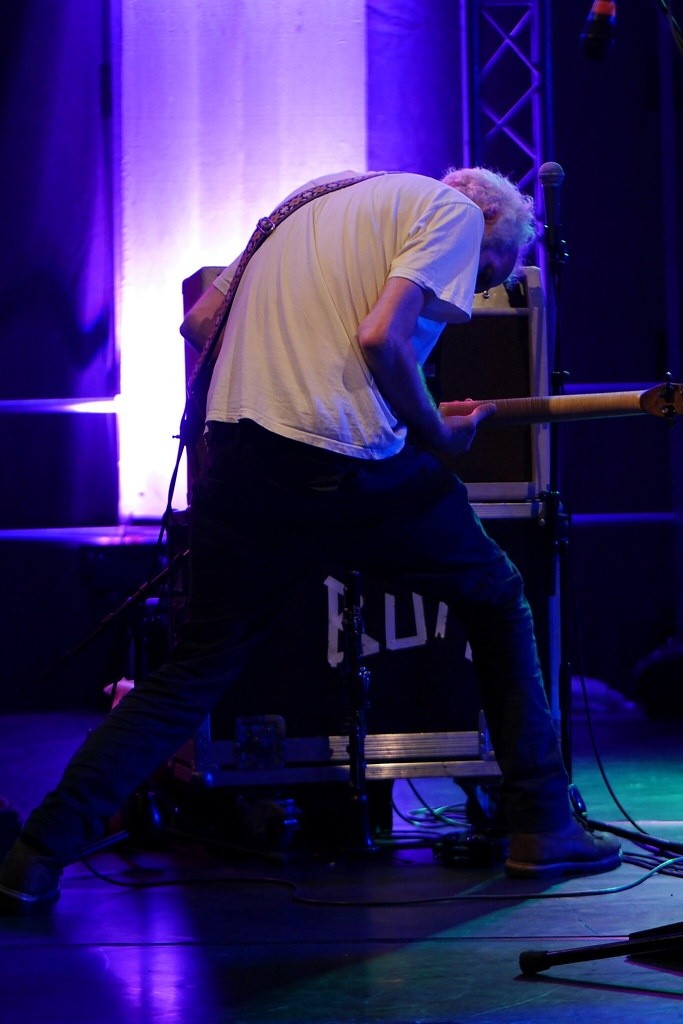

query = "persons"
(0, 166), (626, 915)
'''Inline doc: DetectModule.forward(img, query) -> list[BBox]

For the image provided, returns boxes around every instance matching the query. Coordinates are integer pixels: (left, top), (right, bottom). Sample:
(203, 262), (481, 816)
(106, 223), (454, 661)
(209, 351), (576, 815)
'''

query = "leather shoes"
(0, 834), (62, 913)
(505, 811), (623, 879)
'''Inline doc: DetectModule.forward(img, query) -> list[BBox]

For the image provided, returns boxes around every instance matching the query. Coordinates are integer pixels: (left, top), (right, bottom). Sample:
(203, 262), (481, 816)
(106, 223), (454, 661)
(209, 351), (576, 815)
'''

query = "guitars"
(183, 371), (682, 502)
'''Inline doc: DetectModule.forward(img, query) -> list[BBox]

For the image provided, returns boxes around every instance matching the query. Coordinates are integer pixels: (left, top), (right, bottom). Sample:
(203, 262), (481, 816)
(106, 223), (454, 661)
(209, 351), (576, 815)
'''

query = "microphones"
(585, 0), (618, 43)
(538, 161), (566, 268)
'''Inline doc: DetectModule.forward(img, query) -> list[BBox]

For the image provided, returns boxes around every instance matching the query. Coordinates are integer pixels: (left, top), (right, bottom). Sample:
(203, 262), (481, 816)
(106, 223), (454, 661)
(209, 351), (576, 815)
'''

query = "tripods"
(437, 255), (683, 872)
(62, 549), (335, 871)
